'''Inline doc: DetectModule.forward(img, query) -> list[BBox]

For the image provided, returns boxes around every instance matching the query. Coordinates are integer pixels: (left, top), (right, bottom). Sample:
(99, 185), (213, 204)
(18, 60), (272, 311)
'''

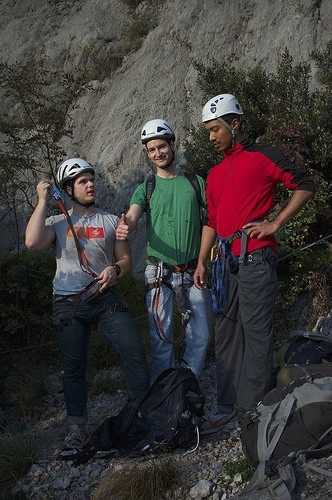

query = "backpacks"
(89, 366), (205, 458)
(240, 336), (332, 496)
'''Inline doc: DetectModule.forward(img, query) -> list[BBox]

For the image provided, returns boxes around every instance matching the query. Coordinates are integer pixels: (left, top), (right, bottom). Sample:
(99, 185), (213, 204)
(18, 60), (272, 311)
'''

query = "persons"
(25, 158), (150, 461)
(193, 94), (315, 436)
(116, 118), (214, 390)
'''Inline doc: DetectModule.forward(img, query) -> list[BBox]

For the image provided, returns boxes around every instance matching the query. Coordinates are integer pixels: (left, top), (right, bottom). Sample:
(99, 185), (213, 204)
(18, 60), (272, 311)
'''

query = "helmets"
(141, 119), (176, 143)
(56, 158), (95, 191)
(202, 94), (244, 123)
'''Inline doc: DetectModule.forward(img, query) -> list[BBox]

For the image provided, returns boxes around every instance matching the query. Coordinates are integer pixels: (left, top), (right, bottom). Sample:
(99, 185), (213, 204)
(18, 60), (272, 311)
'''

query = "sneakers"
(56, 425), (91, 460)
(200, 405), (239, 434)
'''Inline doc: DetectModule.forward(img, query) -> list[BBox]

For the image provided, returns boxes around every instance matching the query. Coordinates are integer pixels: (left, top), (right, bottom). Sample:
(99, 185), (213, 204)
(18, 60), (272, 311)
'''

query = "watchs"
(111, 264), (120, 275)
